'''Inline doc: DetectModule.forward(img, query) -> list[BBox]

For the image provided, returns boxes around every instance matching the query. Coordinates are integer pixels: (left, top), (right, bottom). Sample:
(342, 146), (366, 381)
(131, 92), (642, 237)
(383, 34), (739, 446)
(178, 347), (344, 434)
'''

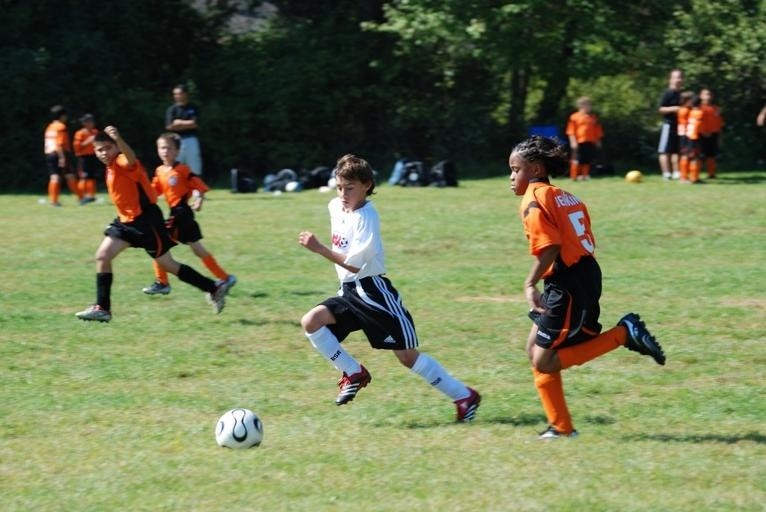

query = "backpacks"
(264, 177), (302, 193)
(430, 161), (458, 186)
(396, 160), (429, 187)
(299, 165), (329, 189)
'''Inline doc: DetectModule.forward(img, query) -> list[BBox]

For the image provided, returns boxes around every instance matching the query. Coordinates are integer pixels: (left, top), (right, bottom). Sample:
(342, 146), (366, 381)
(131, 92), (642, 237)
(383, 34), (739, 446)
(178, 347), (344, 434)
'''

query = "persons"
(299, 154), (482, 423)
(75, 126), (229, 324)
(700, 87), (725, 178)
(677, 89), (694, 184)
(72, 114), (98, 206)
(658, 70), (684, 176)
(687, 97), (704, 182)
(44, 104), (96, 207)
(167, 84), (203, 212)
(567, 96), (603, 181)
(509, 135), (666, 438)
(142, 132), (236, 294)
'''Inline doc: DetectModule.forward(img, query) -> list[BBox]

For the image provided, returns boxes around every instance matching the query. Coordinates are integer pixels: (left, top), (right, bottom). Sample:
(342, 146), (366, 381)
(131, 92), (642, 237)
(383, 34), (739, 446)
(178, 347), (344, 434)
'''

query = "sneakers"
(455, 388), (479, 422)
(209, 284), (226, 310)
(620, 316), (665, 365)
(541, 427), (576, 439)
(77, 305), (112, 323)
(220, 276), (236, 292)
(337, 368), (370, 402)
(144, 282), (169, 295)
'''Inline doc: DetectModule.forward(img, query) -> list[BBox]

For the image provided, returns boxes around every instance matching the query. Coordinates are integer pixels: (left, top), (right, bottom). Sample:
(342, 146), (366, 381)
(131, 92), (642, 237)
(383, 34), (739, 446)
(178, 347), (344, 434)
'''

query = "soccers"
(285, 182), (299, 193)
(329, 178), (337, 189)
(216, 408), (264, 450)
(626, 171), (641, 182)
